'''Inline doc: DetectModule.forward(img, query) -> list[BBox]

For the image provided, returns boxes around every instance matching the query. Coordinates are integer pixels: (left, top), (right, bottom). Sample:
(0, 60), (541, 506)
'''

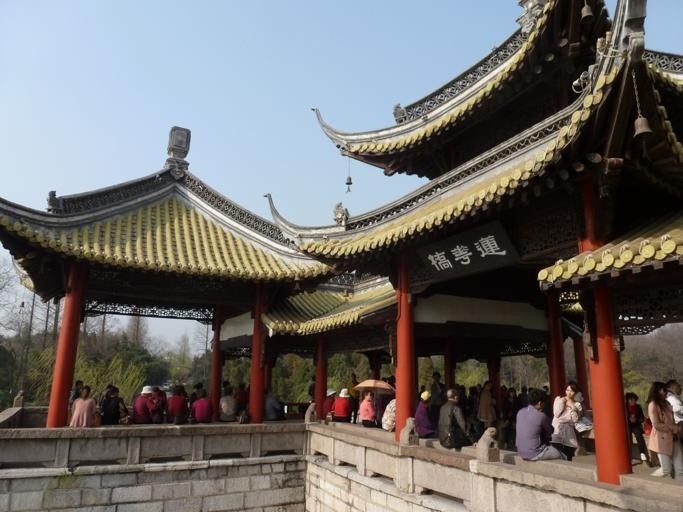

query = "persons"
(308, 375), (316, 403)
(646, 381), (683, 481)
(515, 388), (568, 461)
(549, 380), (584, 461)
(625, 392), (648, 461)
(348, 372), (360, 424)
(322, 388), (337, 420)
(359, 391), (382, 428)
(331, 388), (352, 423)
(381, 371), (534, 449)
(68, 379), (288, 428)
(665, 379), (683, 448)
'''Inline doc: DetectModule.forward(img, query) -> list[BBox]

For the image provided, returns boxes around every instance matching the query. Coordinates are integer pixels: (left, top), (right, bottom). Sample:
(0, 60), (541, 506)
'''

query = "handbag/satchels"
(450, 408), (470, 444)
(118, 415), (131, 424)
(94, 415), (102, 426)
(239, 409), (248, 423)
(644, 419), (652, 433)
(574, 417), (592, 433)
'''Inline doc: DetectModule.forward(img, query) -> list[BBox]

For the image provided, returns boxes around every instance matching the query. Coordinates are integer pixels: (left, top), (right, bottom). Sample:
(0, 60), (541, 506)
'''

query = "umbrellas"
(353, 379), (396, 406)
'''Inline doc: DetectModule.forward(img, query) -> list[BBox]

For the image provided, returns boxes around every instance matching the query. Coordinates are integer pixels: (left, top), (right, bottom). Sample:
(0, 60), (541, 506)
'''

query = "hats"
(326, 388), (336, 396)
(420, 390), (431, 400)
(338, 388), (351, 398)
(140, 386), (152, 394)
